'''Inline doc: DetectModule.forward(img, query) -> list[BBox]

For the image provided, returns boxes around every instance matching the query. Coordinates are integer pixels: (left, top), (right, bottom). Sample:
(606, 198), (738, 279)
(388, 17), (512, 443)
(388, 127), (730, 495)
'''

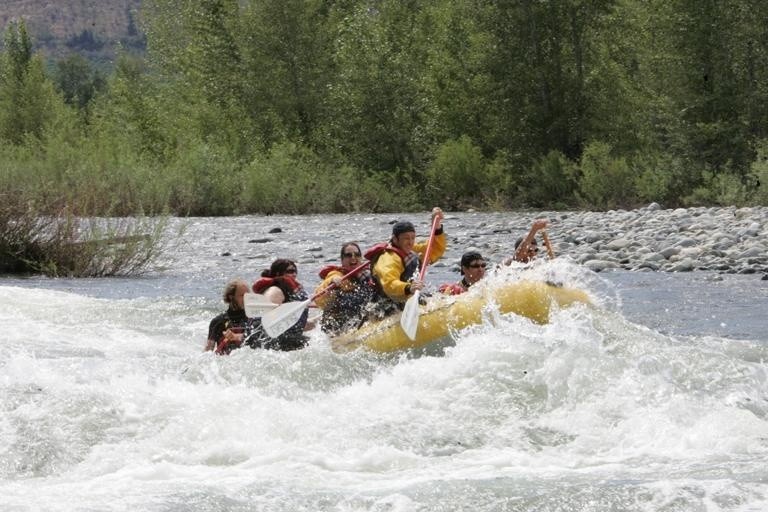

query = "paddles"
(243, 293), (318, 319)
(260, 260), (369, 339)
(401, 214), (440, 340)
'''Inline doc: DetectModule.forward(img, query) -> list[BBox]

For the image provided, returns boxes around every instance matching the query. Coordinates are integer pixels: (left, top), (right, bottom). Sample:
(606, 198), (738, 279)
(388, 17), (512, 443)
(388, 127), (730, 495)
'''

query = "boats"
(309, 283), (593, 367)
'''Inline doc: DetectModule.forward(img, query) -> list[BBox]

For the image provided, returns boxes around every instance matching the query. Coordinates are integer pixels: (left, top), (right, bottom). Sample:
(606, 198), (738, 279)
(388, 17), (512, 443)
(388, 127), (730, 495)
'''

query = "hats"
(461, 251), (480, 275)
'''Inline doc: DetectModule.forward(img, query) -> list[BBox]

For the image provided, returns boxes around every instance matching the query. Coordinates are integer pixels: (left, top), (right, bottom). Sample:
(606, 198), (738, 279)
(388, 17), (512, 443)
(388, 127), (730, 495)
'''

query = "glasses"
(344, 251), (361, 258)
(528, 248), (538, 253)
(286, 268), (297, 274)
(469, 262), (486, 269)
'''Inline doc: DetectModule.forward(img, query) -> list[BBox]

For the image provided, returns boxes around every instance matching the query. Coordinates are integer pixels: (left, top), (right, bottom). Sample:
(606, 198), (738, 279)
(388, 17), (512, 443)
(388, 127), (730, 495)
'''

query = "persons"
(262, 259), (309, 335)
(313, 241), (373, 338)
(440, 251), (488, 296)
(202, 279), (251, 354)
(368, 206), (444, 316)
(502, 218), (551, 266)
